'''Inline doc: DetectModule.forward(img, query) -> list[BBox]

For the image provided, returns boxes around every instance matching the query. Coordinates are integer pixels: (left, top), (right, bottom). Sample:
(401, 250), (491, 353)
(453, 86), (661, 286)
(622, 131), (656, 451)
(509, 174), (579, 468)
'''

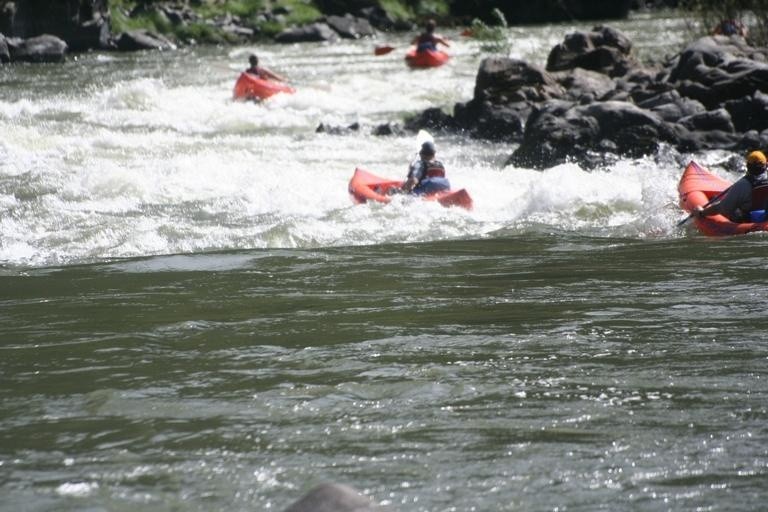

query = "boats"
(714, 20), (748, 36)
(348, 167), (474, 213)
(234, 72), (295, 100)
(374, 30), (475, 67)
(678, 160), (768, 236)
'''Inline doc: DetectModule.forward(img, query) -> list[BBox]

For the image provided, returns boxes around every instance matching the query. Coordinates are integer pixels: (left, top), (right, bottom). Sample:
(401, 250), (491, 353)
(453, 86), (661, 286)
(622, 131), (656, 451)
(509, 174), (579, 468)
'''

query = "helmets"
(419, 141), (435, 155)
(745, 150), (766, 168)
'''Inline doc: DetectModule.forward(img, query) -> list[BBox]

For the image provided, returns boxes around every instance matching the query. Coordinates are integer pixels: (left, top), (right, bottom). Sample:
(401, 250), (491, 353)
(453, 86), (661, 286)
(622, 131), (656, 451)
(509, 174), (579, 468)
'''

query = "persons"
(690, 149), (768, 219)
(388, 141), (446, 196)
(410, 19), (452, 54)
(245, 54), (289, 84)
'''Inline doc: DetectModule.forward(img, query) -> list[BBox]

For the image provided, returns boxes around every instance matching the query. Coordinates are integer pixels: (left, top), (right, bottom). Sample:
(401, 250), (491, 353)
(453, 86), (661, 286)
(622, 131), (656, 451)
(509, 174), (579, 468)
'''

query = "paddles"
(374, 29), (475, 55)
